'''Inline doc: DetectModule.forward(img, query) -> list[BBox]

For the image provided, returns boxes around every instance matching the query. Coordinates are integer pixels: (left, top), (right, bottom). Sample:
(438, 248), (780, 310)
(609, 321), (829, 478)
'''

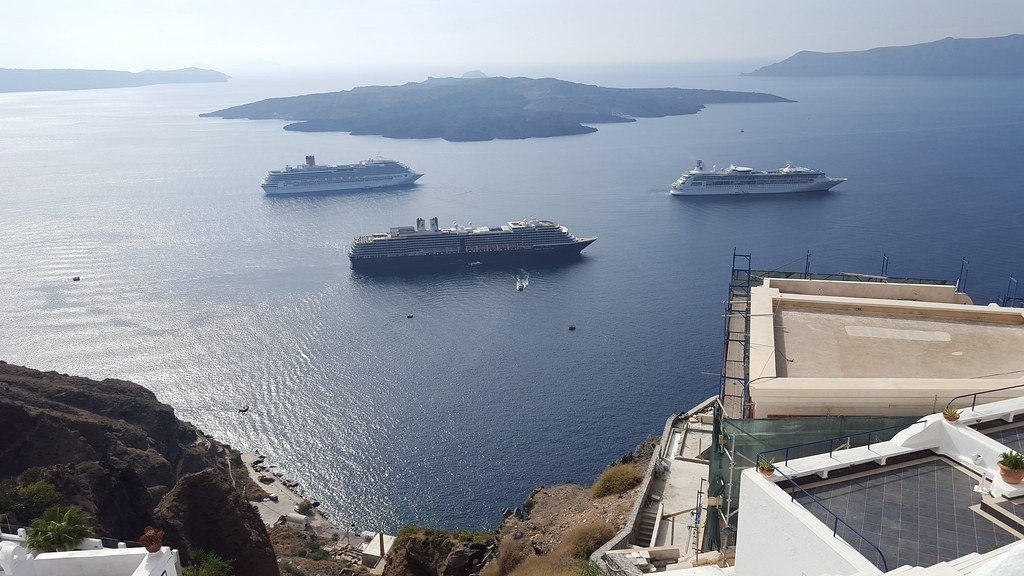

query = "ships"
(347, 217), (598, 271)
(670, 160), (848, 195)
(260, 156), (424, 195)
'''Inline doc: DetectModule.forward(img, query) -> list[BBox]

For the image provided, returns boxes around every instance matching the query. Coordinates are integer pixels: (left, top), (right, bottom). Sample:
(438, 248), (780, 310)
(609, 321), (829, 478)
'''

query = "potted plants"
(996, 450), (1024, 484)
(757, 457), (780, 476)
(943, 404), (963, 421)
(139, 526), (165, 553)
